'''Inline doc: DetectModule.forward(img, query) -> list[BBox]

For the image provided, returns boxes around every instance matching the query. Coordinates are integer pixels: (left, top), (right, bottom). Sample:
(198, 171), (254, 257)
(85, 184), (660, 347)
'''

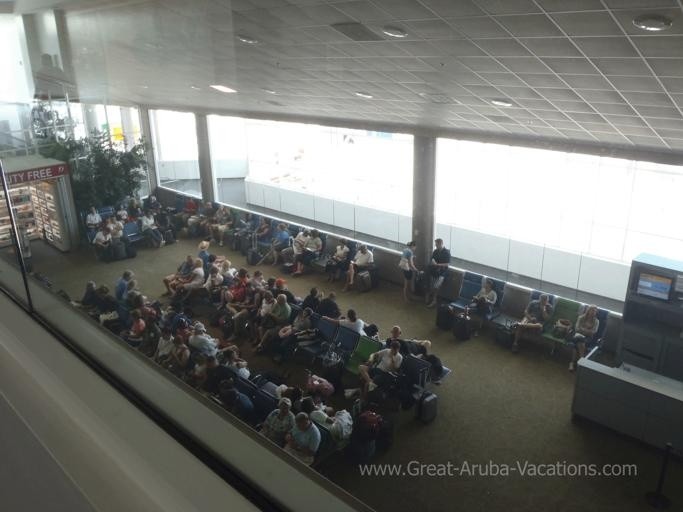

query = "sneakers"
(367, 382), (378, 392)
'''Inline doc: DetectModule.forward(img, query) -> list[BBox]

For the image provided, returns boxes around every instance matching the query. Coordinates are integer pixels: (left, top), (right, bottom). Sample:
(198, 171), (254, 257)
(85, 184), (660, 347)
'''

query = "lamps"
(632, 15), (673, 33)
(382, 25), (407, 38)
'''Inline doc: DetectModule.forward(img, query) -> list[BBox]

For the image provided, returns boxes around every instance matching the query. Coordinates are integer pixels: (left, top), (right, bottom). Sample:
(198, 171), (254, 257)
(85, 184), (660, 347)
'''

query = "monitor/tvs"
(637, 269), (673, 300)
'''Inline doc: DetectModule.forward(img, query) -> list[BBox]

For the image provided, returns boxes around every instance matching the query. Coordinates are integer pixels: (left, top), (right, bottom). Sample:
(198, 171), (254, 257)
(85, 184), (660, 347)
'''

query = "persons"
(27, 193), (450, 467)
(509, 293), (553, 353)
(568, 305), (599, 373)
(464, 279), (498, 338)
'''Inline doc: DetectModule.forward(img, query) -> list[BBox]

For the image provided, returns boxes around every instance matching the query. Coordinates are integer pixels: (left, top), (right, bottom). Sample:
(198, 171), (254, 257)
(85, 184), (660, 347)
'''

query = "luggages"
(247, 231), (258, 265)
(453, 306), (471, 339)
(436, 303), (454, 330)
(498, 320), (513, 347)
(356, 270), (372, 291)
(415, 367), (437, 422)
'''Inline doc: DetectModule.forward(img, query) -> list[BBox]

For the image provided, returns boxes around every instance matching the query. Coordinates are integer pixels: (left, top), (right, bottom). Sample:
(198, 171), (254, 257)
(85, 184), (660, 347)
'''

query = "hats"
(277, 398), (291, 408)
(389, 341), (400, 349)
(129, 309), (141, 318)
(199, 240), (210, 251)
(276, 279), (287, 287)
(195, 323), (206, 332)
(268, 277), (276, 283)
(260, 291), (277, 298)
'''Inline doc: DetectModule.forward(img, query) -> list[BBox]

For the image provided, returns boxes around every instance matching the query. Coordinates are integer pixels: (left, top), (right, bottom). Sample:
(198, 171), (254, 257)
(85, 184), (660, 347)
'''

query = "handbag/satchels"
(353, 410), (385, 440)
(113, 241), (136, 259)
(553, 317), (573, 337)
(424, 355), (442, 376)
(399, 258), (410, 271)
(309, 375), (335, 399)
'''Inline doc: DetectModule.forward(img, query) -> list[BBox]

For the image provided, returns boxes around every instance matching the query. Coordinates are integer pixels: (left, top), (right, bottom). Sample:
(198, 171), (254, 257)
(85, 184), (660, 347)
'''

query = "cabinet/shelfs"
(0, 179), (71, 252)
